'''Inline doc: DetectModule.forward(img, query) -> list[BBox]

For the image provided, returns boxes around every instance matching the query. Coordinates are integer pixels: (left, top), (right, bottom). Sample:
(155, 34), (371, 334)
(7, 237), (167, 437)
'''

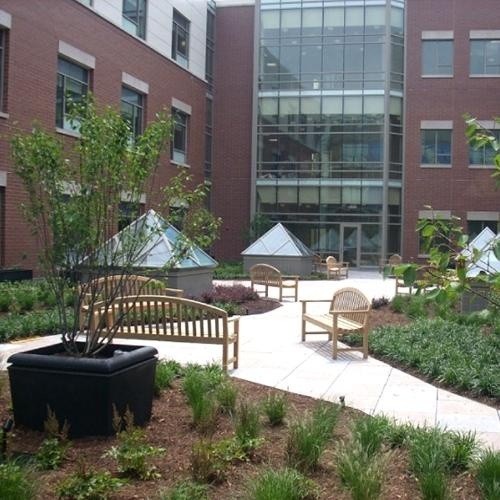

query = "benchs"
(73, 276), (183, 335)
(82, 294), (240, 372)
(313, 254), (327, 274)
(326, 256), (351, 278)
(249, 263), (300, 301)
(395, 266), (446, 296)
(380, 254), (402, 279)
(299, 285), (373, 360)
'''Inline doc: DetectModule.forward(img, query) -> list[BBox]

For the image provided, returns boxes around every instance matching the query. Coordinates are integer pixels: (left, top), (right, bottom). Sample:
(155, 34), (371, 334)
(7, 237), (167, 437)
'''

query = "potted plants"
(6, 90), (222, 434)
